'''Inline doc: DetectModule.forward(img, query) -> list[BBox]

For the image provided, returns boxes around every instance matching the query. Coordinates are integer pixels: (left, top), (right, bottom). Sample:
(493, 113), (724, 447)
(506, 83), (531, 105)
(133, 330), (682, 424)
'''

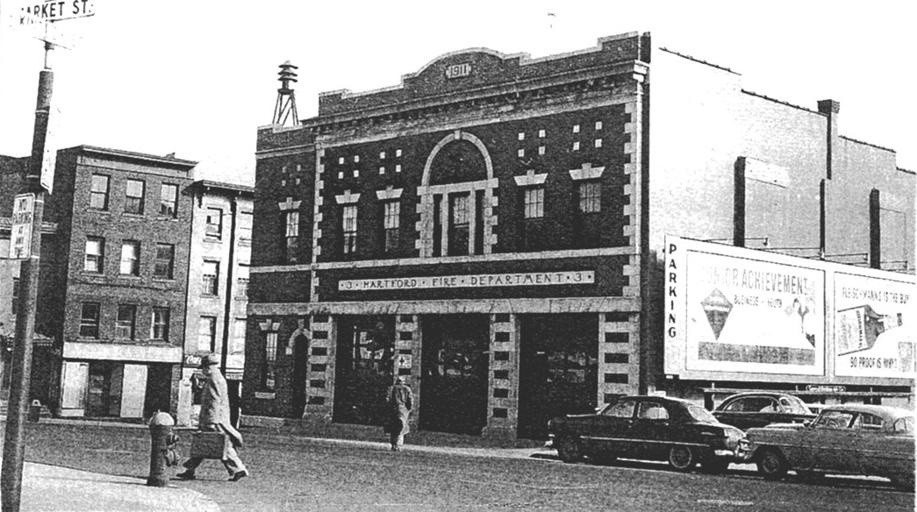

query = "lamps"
(703, 237), (915, 271)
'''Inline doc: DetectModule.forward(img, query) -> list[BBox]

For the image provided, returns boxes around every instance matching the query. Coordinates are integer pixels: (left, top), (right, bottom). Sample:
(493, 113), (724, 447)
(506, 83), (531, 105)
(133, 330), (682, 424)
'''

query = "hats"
(197, 354), (220, 370)
(395, 376), (405, 382)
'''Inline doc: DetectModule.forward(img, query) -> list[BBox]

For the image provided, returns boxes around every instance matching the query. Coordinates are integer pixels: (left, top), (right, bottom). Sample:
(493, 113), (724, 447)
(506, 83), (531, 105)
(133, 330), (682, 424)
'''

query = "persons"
(176, 354), (249, 482)
(384, 376), (414, 451)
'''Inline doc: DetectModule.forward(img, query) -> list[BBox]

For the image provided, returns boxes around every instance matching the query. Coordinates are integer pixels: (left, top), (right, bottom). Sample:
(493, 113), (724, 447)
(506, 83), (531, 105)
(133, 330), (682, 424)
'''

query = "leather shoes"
(228, 471), (246, 481)
(391, 447), (398, 451)
(176, 472), (196, 479)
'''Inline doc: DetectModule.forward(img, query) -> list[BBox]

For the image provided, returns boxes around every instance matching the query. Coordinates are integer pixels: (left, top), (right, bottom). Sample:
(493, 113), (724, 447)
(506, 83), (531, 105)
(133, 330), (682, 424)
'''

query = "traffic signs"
(11, 9), (77, 56)
(1, 0), (97, 26)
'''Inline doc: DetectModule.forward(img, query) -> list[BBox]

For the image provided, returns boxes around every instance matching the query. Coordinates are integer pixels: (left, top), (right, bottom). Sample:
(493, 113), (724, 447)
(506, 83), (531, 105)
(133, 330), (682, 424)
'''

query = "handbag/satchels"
(189, 428), (224, 459)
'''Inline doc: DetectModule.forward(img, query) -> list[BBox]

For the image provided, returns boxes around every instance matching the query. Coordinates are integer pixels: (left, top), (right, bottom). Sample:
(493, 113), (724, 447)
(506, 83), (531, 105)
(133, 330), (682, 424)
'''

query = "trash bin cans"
(29, 399), (42, 422)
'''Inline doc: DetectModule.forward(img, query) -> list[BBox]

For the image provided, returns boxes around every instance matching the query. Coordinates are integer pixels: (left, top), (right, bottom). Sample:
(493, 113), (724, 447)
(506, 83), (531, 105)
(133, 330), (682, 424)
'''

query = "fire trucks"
(146, 410), (184, 488)
